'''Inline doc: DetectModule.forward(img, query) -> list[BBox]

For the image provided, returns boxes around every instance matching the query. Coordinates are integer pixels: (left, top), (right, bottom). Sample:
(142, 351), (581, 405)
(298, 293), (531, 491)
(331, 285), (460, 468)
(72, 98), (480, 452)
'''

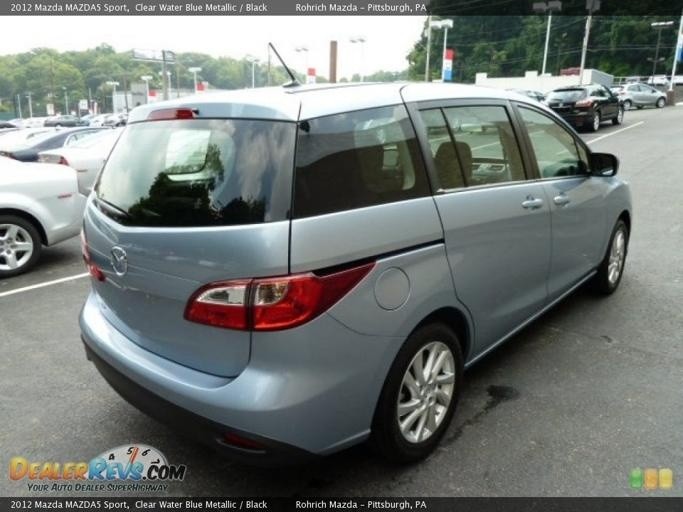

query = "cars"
(0, 74), (683, 466)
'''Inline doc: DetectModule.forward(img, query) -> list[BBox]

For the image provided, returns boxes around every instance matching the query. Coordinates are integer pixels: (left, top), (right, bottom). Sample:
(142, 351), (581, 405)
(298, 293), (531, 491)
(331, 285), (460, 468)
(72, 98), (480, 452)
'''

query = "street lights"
(647, 21), (674, 73)
(432, 19), (453, 82)
(579, 0), (601, 84)
(533, 0), (562, 75)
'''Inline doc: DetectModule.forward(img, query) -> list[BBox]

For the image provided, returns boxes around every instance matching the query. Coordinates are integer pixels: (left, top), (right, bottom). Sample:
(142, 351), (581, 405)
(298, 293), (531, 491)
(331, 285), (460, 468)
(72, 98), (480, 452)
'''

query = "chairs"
(354, 129), (384, 186)
(433, 141), (473, 190)
